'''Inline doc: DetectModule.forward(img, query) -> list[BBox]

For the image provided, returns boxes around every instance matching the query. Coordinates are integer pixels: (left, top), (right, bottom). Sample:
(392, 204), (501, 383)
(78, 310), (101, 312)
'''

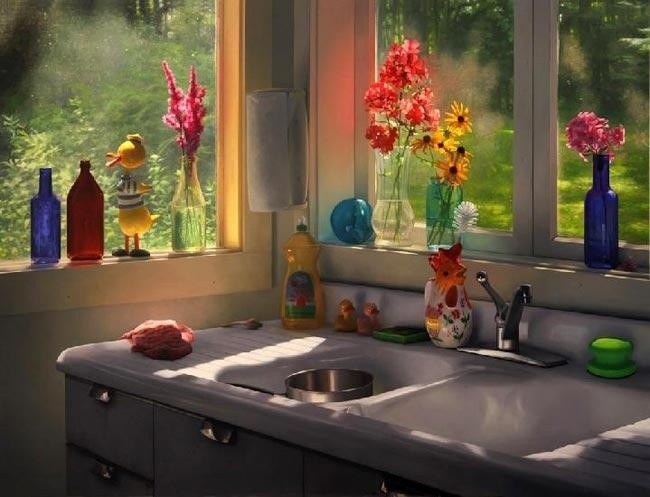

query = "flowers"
(162, 60), (209, 245)
(565, 110), (626, 164)
(361, 39), (473, 247)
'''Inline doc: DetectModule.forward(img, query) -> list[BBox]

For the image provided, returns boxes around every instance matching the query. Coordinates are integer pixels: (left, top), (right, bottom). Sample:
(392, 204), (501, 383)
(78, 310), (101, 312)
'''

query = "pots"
(225, 367), (375, 404)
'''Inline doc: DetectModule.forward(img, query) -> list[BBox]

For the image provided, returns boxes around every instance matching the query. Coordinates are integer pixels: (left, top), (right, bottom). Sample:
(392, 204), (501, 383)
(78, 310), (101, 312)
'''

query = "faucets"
(477, 270), (532, 350)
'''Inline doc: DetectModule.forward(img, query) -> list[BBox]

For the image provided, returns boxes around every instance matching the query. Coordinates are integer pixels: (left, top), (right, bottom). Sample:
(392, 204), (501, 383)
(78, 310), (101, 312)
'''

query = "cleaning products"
(281, 216), (324, 328)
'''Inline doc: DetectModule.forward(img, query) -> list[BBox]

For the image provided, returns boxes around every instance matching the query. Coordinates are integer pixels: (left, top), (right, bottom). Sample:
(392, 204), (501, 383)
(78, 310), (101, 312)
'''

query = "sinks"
(216, 345), (457, 405)
(335, 369), (650, 460)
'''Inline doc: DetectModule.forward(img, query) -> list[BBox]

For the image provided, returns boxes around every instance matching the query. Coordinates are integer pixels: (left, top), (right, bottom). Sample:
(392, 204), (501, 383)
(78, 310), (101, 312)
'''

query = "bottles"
(66, 159), (105, 261)
(30, 167), (62, 265)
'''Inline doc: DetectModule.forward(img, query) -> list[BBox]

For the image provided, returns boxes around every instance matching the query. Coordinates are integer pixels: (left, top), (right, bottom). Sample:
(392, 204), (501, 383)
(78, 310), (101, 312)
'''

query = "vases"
(30, 160), (104, 264)
(370, 148), (464, 253)
